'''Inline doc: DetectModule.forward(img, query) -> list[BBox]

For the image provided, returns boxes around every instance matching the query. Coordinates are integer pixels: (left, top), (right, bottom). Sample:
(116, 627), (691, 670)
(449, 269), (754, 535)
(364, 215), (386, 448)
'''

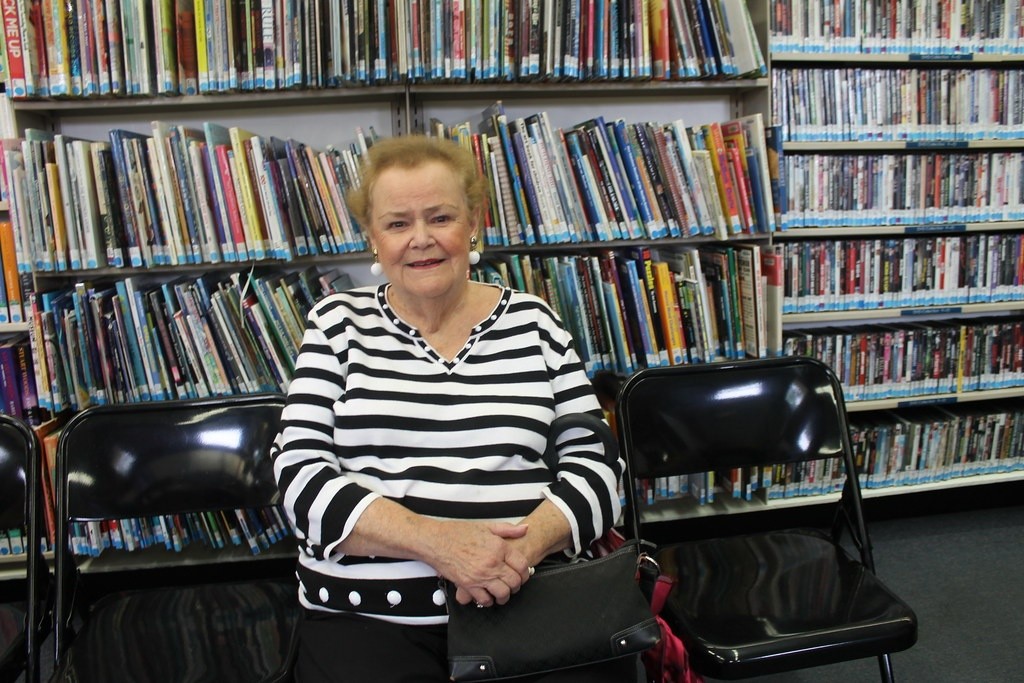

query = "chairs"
(0, 413), (55, 683)
(46, 393), (302, 683)
(615, 356), (918, 683)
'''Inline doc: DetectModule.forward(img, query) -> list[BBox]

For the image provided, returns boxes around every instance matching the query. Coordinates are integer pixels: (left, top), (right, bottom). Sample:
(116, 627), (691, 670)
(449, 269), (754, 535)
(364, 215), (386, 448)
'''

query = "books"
(0, 0), (1024, 555)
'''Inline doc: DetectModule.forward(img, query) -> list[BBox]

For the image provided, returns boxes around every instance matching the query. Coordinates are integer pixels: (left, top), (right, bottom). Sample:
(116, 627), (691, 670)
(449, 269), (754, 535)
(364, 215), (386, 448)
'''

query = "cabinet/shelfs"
(0, 1), (1024, 586)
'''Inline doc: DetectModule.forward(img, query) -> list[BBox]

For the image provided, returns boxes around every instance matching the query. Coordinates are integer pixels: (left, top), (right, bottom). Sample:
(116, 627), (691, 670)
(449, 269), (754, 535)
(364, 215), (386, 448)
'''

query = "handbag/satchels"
(439, 539), (664, 683)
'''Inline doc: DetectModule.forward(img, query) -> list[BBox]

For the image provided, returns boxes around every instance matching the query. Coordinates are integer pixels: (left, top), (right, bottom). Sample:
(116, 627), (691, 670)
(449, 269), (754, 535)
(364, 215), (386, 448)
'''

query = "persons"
(270, 137), (627, 683)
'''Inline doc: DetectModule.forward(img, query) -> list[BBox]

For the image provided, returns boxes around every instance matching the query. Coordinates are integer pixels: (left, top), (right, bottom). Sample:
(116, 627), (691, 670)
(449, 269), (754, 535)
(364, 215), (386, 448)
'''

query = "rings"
(476, 601), (483, 608)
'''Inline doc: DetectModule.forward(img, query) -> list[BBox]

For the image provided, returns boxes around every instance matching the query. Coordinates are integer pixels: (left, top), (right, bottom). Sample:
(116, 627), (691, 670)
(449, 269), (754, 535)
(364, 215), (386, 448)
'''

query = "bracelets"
(527, 567), (535, 575)
